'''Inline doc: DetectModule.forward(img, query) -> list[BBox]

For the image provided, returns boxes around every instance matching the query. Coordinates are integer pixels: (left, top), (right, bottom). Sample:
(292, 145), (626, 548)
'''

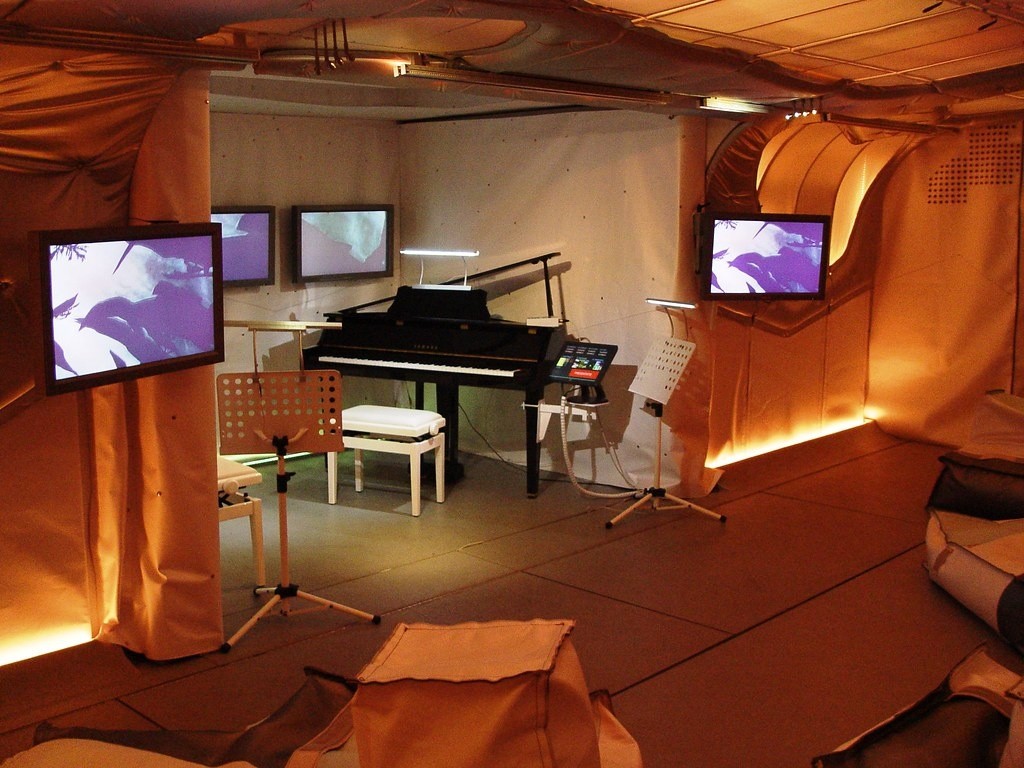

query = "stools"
(324, 404), (447, 517)
(215, 455), (266, 598)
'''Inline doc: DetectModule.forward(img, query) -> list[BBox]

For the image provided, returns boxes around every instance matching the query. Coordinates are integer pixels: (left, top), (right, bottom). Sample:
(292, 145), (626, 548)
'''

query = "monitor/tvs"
(26, 222), (224, 396)
(700, 212), (831, 300)
(208, 205), (276, 288)
(291, 204), (394, 283)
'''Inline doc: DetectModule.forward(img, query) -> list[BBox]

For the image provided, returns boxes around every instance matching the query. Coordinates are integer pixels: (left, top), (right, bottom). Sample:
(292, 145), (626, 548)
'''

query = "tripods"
(215, 369), (380, 654)
(604, 338), (727, 529)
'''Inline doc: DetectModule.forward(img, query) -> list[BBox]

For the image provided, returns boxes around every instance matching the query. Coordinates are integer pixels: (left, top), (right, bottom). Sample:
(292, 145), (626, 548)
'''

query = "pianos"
(299, 286), (567, 498)
